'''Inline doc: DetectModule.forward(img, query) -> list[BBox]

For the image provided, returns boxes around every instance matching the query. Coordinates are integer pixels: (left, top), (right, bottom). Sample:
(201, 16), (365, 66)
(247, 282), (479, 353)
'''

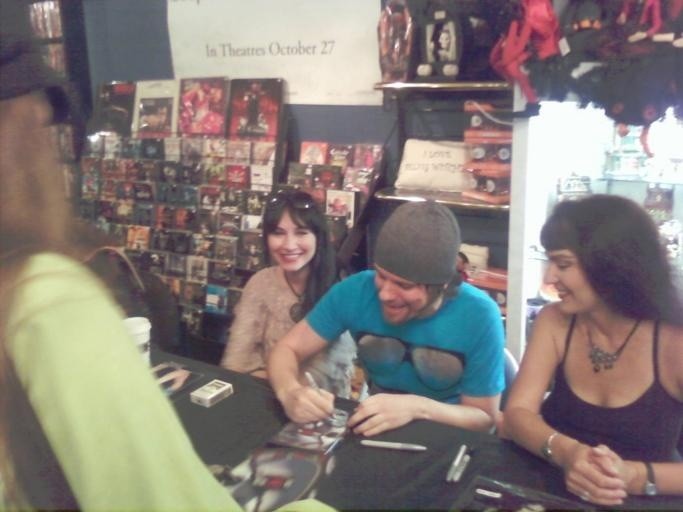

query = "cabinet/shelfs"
(373, 79), (512, 294)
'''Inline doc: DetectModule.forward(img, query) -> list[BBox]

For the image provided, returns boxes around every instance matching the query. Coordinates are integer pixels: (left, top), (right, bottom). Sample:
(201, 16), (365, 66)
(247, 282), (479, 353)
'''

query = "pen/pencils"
(305, 371), (337, 420)
(445, 444), (470, 482)
(360, 440), (427, 451)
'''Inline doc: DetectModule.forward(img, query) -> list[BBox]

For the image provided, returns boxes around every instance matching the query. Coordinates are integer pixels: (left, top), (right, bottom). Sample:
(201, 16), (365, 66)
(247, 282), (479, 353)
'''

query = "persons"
(0, 25), (337, 512)
(542, 432), (557, 470)
(502, 194), (683, 505)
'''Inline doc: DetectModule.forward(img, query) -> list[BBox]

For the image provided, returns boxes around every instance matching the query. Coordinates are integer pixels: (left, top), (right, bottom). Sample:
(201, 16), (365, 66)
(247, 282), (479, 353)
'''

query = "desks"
(151, 345), (683, 511)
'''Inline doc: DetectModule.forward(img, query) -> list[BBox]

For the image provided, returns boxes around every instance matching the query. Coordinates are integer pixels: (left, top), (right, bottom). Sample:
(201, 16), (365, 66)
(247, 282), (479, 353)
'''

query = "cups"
(123, 315), (152, 368)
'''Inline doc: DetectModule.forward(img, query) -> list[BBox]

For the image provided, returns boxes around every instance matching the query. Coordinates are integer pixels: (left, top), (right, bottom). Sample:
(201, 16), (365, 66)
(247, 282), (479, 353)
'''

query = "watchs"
(641, 458), (657, 501)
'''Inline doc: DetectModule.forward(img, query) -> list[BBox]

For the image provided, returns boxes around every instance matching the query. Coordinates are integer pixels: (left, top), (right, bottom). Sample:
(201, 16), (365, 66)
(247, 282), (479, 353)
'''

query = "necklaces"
(585, 315), (642, 372)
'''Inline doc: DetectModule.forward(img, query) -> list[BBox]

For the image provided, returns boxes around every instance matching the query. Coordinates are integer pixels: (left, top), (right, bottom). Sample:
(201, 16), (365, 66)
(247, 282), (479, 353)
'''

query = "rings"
(581, 491), (591, 502)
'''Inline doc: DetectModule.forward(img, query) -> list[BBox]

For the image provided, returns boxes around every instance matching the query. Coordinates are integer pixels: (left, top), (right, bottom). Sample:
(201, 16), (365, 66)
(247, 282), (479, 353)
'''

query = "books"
(151, 363), (205, 398)
(266, 407), (356, 455)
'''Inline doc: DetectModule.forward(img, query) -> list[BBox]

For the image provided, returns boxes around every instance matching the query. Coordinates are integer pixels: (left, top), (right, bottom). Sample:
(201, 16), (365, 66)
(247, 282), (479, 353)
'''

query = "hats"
(373, 199), (460, 284)
(0, 0), (70, 122)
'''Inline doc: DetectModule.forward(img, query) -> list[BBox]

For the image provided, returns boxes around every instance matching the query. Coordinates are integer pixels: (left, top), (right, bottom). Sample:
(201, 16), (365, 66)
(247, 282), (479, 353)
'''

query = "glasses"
(357, 331), (464, 389)
(266, 193), (315, 210)
(53, 122), (87, 165)
